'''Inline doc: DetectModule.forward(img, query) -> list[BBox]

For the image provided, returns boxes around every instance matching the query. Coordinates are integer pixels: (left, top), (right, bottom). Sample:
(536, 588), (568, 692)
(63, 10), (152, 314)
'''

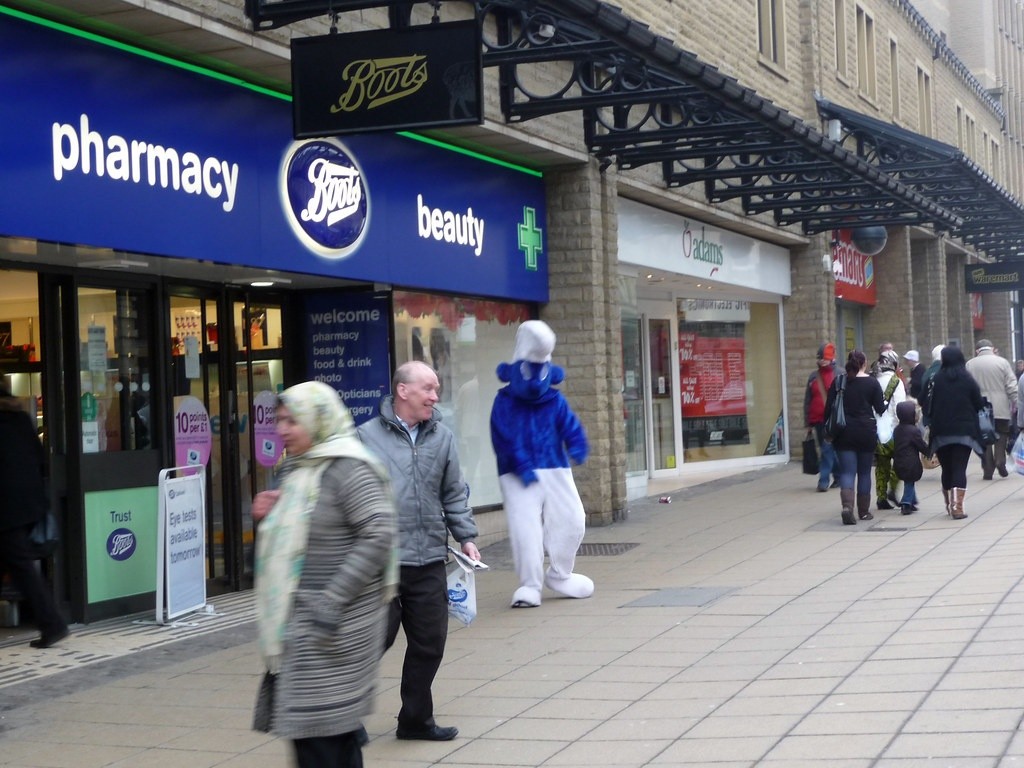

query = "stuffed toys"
(490, 319), (594, 607)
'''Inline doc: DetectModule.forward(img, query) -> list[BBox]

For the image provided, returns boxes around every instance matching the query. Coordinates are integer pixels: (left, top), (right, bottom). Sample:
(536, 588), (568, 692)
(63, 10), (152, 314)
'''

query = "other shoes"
(816, 482), (829, 492)
(830, 479), (840, 488)
(997, 464), (1009, 478)
(887, 494), (899, 507)
(901, 504), (912, 515)
(29, 626), (70, 648)
(982, 463), (993, 480)
(395, 723), (458, 741)
(877, 500), (895, 509)
(910, 503), (917, 511)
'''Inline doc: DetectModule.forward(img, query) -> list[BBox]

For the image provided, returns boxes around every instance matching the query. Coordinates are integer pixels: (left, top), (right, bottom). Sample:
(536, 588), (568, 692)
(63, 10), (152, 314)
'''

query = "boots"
(942, 488), (968, 519)
(857, 494), (873, 520)
(841, 489), (856, 525)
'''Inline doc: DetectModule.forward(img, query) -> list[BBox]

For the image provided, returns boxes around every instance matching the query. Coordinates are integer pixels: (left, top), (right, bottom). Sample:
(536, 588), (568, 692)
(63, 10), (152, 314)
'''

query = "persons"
(1, 372), (69, 647)
(803, 339), (1024, 525)
(354, 361), (480, 747)
(251, 380), (400, 768)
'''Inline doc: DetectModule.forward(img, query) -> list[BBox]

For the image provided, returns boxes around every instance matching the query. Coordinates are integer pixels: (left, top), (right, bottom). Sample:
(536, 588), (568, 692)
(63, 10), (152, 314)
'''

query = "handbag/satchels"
(801, 431), (819, 474)
(822, 374), (847, 443)
(1007, 432), (1024, 476)
(921, 429), (941, 469)
(444, 564), (477, 625)
(1006, 412), (1021, 454)
(978, 407), (999, 445)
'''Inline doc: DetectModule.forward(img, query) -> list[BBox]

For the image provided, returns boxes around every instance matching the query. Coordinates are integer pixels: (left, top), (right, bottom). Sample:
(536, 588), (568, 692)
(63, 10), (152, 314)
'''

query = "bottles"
(251, 322), (263, 349)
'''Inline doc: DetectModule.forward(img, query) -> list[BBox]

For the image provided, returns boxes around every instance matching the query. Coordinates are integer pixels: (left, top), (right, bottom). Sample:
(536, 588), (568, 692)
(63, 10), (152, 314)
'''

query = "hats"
(816, 343), (835, 360)
(974, 339), (993, 353)
(902, 350), (919, 362)
(931, 344), (946, 362)
